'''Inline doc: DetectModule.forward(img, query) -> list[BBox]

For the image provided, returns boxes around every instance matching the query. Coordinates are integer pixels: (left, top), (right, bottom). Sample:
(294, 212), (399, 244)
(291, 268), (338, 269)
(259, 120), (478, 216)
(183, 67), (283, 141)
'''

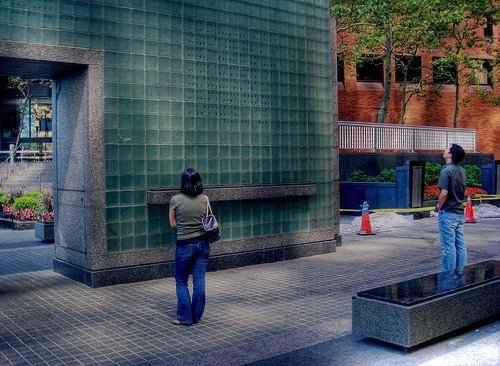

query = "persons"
(436, 143), (467, 293)
(168, 167), (210, 325)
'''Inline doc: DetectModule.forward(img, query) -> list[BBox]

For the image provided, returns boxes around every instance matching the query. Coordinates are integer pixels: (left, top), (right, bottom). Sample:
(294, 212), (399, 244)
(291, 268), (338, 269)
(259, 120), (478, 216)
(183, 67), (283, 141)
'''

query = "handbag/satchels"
(202, 195), (221, 242)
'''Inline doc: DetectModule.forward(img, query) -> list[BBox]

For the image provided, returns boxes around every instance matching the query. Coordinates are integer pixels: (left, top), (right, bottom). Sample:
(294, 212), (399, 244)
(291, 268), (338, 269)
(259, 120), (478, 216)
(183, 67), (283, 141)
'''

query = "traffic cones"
(356, 201), (377, 235)
(462, 194), (477, 223)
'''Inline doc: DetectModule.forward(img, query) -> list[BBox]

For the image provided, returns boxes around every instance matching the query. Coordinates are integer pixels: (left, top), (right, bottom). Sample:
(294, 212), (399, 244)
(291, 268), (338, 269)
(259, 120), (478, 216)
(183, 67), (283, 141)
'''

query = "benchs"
(35, 222), (55, 243)
(352, 259), (500, 352)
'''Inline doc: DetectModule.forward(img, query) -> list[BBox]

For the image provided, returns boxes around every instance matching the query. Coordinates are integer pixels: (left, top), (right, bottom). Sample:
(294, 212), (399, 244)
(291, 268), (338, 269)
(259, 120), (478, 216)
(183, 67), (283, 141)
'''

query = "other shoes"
(172, 319), (180, 324)
